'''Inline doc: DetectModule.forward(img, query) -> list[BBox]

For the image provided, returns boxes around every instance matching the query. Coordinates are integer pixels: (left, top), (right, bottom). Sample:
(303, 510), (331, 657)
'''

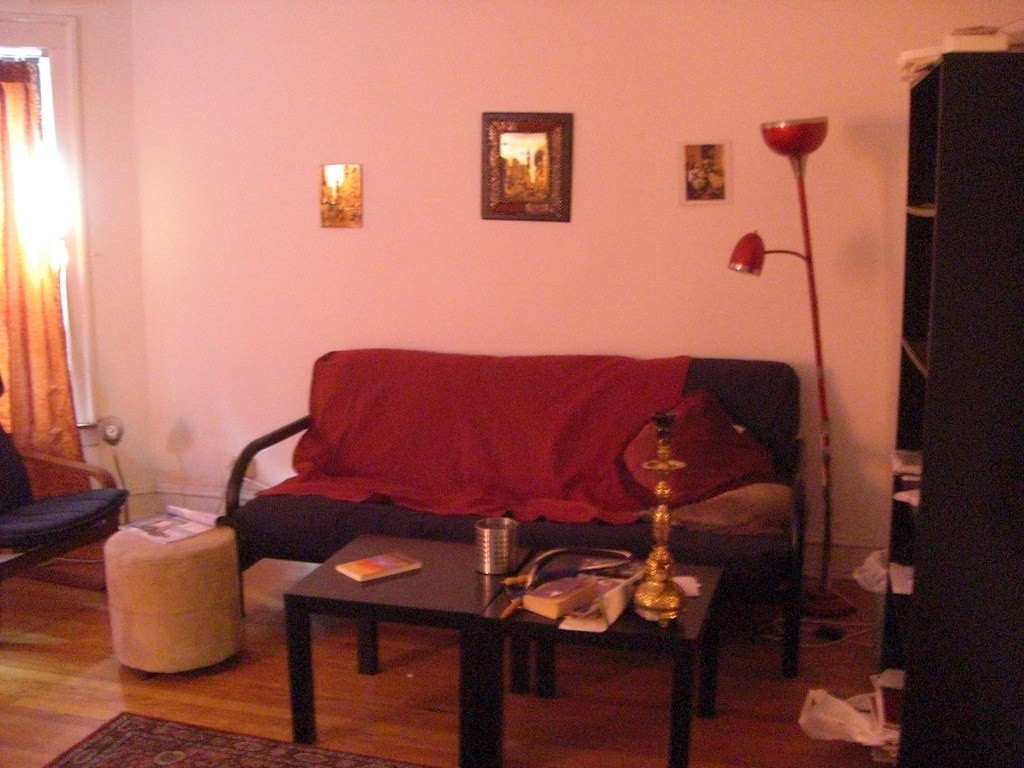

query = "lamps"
(728, 115), (858, 618)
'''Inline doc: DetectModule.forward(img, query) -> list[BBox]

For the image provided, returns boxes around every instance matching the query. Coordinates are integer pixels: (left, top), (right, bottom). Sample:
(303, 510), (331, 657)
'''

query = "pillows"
(612, 383), (778, 506)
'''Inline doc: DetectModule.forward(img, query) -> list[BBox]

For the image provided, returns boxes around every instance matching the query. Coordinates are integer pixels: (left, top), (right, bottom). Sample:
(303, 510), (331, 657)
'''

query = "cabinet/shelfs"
(870, 52), (1024, 768)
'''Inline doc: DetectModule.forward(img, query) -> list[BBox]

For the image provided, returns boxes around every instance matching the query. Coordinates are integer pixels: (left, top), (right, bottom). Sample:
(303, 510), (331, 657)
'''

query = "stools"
(109, 527), (245, 679)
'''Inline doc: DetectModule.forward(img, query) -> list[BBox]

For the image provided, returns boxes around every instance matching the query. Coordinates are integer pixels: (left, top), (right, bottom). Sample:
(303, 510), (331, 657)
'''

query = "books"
(118, 505), (225, 545)
(336, 551), (423, 583)
(890, 563), (913, 593)
(522, 567), (645, 633)
(890, 450), (920, 474)
(942, 22), (1012, 50)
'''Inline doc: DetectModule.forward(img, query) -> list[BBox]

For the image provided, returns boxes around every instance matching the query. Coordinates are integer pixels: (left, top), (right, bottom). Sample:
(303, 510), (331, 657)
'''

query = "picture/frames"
(480, 111), (574, 223)
(682, 142), (731, 202)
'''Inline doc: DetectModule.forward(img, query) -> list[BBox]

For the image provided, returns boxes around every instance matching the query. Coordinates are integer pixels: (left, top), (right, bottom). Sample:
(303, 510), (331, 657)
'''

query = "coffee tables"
(283, 536), (725, 768)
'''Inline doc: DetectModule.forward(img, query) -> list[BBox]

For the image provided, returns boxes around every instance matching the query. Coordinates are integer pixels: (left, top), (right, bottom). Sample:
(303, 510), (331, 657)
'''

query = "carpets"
(41, 711), (425, 768)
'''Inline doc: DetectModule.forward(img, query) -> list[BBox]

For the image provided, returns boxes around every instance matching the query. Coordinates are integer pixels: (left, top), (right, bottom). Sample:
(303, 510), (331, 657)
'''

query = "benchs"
(208, 348), (811, 676)
(0, 400), (127, 580)
